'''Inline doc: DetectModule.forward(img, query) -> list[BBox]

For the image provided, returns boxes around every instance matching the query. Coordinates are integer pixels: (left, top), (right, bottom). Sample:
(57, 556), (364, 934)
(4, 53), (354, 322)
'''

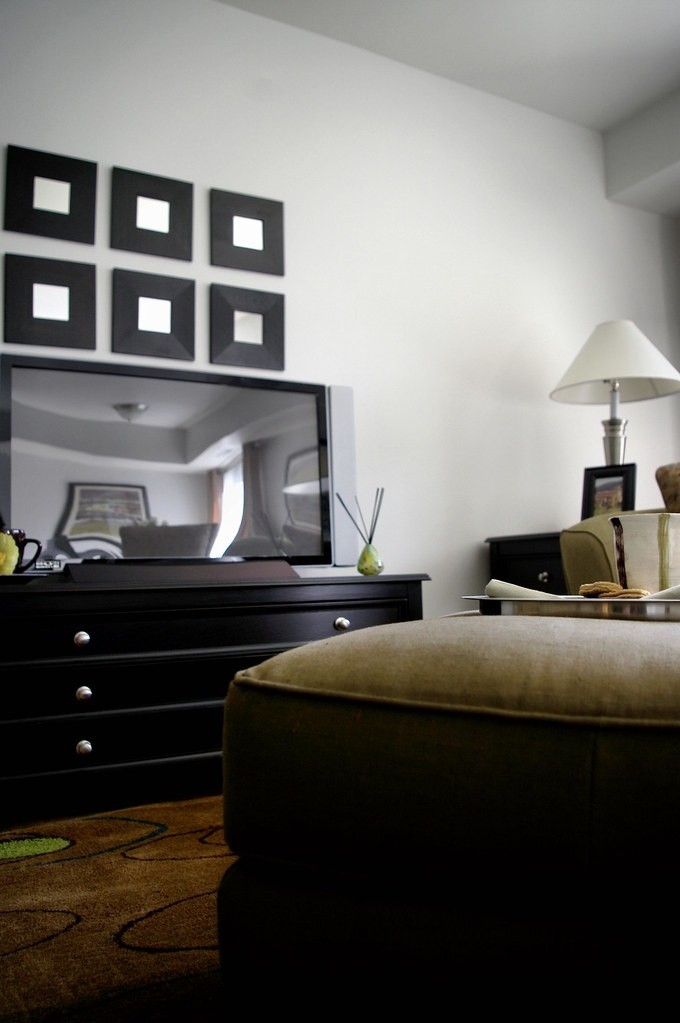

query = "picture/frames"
(581, 461), (638, 523)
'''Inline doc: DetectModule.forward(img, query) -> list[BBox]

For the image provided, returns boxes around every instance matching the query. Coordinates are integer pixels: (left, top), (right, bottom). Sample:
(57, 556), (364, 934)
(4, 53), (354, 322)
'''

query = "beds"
(218, 615), (679, 1023)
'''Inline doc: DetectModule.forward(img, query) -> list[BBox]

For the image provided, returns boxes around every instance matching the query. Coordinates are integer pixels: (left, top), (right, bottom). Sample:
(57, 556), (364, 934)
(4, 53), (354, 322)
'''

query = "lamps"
(550, 318), (679, 466)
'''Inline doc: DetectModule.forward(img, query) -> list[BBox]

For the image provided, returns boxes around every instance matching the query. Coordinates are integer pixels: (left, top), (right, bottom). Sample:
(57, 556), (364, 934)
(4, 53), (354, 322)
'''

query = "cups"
(2, 528), (43, 574)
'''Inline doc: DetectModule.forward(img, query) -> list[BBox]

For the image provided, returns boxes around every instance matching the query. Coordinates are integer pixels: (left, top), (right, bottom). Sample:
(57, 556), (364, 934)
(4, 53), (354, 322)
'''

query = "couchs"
(559, 507), (667, 594)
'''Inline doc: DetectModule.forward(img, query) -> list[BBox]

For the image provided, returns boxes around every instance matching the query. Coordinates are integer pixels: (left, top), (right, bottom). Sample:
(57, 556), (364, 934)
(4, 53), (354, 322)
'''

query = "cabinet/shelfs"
(0, 567), (433, 831)
(485, 525), (566, 605)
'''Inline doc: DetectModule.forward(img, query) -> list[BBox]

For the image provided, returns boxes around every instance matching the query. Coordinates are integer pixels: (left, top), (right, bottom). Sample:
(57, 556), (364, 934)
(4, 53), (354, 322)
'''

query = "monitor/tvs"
(0, 353), (333, 583)
